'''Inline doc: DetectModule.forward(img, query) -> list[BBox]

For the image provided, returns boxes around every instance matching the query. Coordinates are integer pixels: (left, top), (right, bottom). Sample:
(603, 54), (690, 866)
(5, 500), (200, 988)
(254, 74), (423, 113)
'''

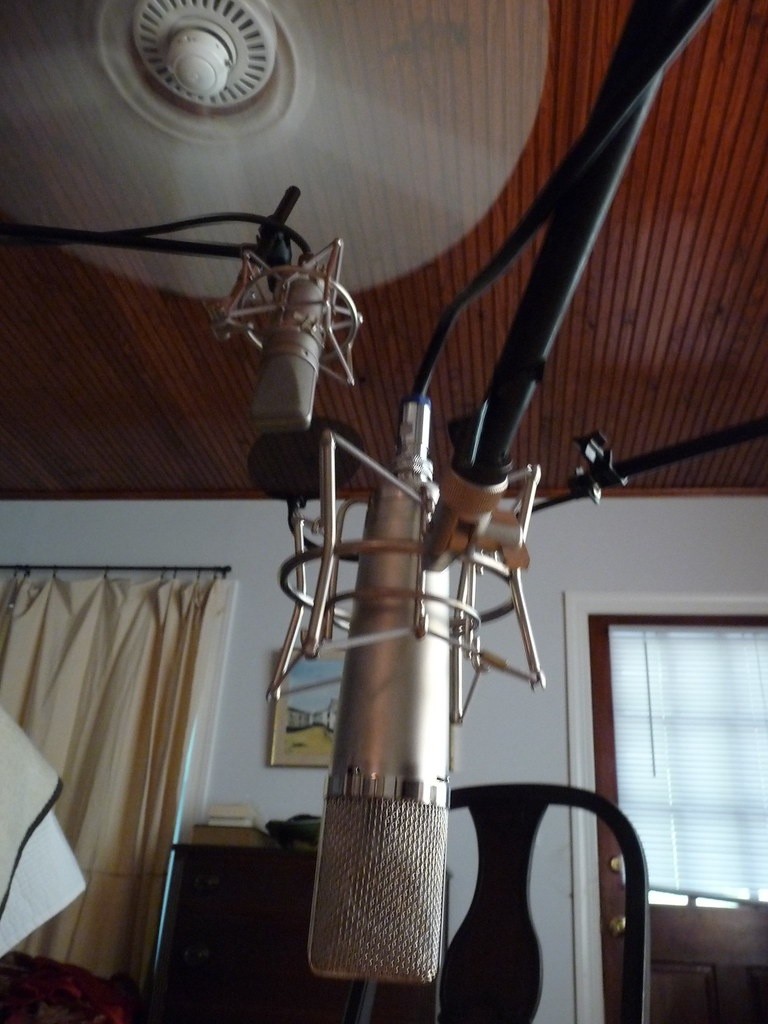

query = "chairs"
(334, 783), (653, 1021)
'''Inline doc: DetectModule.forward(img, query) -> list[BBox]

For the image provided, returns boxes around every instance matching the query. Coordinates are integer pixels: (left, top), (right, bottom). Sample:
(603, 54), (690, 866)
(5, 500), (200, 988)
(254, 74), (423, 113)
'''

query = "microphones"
(309, 395), (451, 984)
(248, 262), (329, 433)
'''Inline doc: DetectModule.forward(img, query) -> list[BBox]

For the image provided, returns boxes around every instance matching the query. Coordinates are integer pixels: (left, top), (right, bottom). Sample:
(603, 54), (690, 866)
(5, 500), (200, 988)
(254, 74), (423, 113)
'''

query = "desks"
(166, 842), (362, 1022)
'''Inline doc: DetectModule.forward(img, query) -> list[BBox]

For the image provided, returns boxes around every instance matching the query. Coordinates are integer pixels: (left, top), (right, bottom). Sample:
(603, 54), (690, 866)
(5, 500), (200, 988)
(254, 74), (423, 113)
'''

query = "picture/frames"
(270, 647), (346, 768)
